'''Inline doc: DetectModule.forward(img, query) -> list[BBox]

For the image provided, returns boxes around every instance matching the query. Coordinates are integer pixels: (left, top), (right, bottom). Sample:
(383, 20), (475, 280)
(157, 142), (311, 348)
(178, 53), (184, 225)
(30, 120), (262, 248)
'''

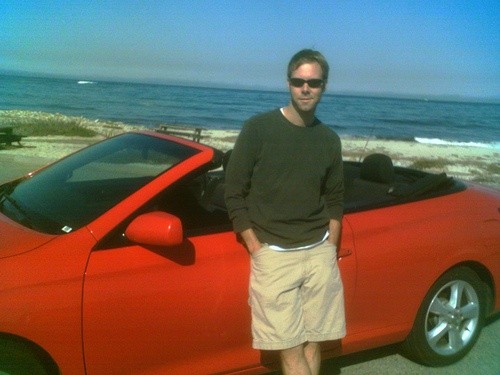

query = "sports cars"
(0, 129), (500, 375)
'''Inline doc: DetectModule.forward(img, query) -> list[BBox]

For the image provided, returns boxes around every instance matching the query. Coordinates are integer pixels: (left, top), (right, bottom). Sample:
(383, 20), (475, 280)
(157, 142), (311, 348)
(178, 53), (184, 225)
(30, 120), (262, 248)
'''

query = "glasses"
(289, 78), (325, 88)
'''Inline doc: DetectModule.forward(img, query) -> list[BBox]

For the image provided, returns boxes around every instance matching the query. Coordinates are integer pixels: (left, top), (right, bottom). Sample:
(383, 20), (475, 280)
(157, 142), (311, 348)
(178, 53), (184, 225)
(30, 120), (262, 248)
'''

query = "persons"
(224, 48), (346, 375)
